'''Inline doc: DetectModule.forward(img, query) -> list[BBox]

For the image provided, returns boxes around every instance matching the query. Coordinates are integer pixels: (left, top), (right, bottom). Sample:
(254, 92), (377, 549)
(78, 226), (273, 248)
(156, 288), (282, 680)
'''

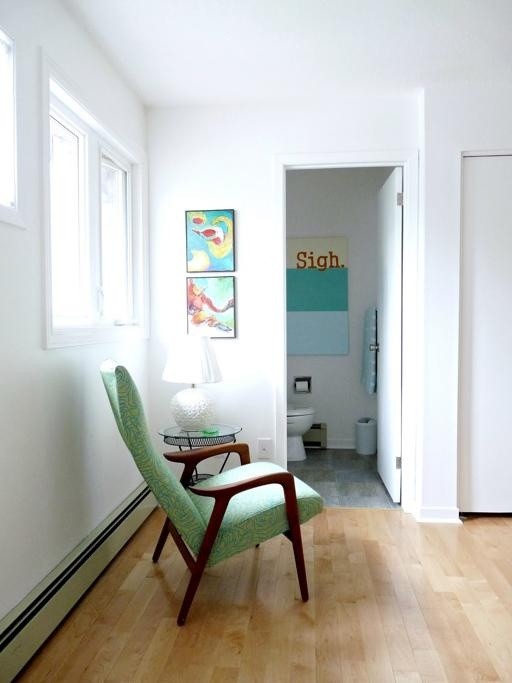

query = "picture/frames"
(185, 209), (235, 272)
(187, 277), (237, 339)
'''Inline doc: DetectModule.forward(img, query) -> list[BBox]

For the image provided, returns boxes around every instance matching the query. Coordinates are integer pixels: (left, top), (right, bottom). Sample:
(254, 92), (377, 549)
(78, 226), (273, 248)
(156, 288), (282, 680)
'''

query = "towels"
(360, 307), (376, 394)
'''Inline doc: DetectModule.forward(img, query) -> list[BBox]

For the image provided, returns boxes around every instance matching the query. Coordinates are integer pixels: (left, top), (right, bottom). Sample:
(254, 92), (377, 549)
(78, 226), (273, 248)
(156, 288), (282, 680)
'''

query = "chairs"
(96, 356), (325, 625)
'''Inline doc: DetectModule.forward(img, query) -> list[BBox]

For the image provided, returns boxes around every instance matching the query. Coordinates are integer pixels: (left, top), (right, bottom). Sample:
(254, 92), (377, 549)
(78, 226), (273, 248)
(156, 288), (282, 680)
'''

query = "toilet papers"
(296, 381), (309, 392)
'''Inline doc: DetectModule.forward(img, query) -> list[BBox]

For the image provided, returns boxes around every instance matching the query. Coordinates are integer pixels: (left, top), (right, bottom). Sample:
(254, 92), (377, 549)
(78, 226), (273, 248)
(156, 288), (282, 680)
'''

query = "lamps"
(163, 336), (223, 432)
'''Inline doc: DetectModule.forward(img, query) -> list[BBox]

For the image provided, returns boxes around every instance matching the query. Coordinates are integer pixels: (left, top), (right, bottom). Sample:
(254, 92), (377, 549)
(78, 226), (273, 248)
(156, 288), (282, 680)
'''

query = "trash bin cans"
(356, 417), (376, 455)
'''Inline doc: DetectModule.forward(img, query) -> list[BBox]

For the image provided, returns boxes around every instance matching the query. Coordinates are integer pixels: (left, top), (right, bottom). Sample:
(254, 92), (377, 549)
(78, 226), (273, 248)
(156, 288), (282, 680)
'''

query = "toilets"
(287, 405), (315, 462)
